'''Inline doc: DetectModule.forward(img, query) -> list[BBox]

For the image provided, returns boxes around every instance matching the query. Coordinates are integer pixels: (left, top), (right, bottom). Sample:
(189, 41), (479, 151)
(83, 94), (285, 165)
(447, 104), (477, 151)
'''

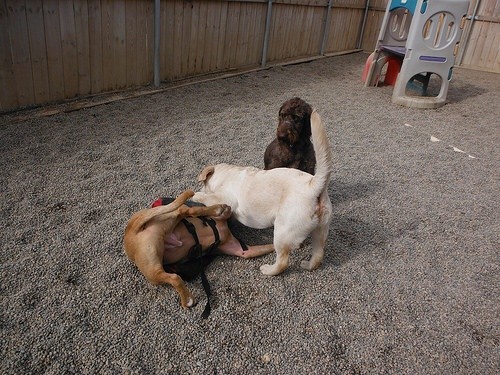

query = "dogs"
(123, 96), (333, 311)
